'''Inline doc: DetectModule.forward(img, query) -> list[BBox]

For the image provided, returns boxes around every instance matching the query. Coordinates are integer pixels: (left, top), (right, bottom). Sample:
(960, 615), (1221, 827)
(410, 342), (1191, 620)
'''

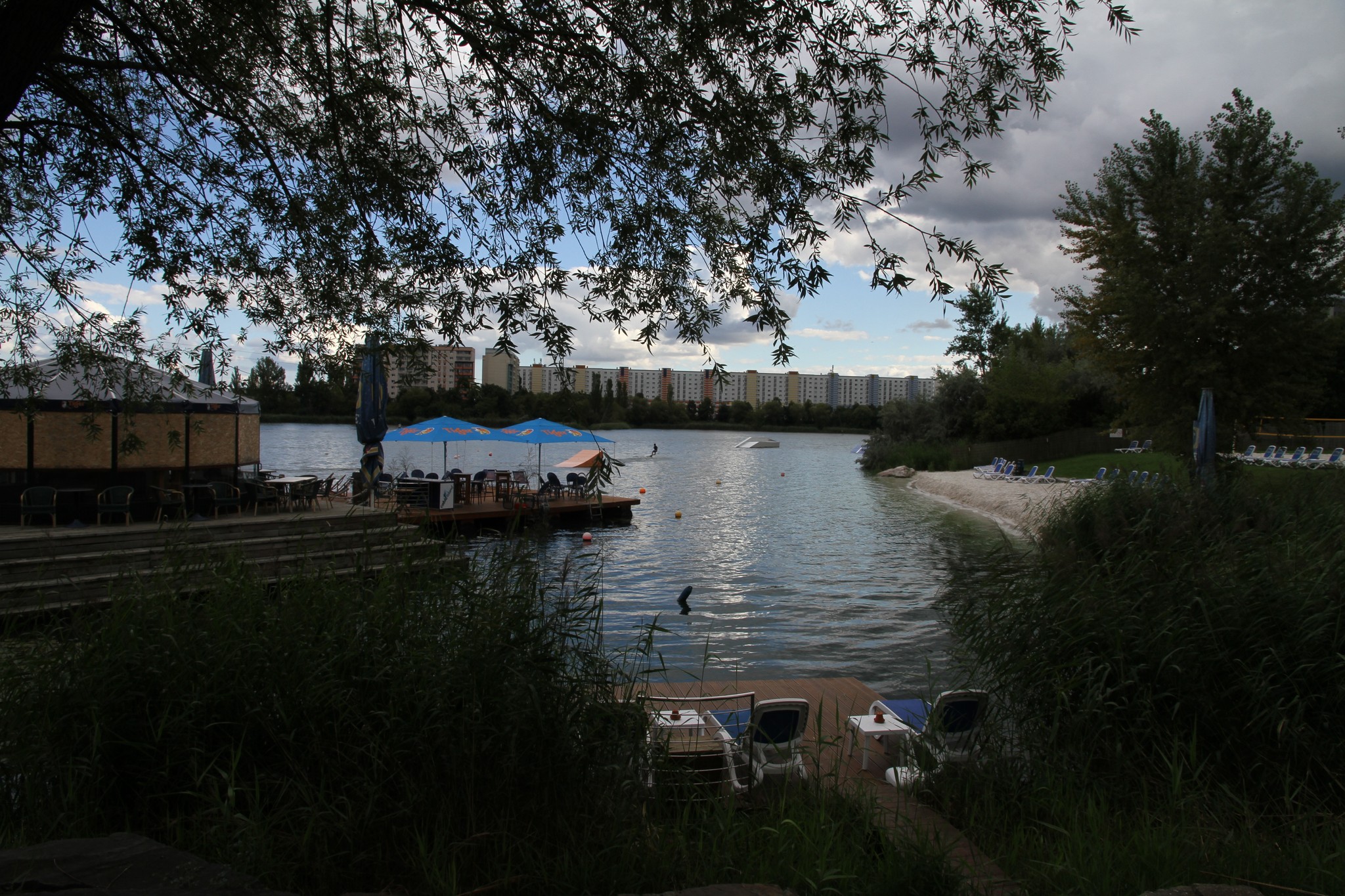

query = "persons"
(649, 443), (658, 457)
(398, 423), (401, 429)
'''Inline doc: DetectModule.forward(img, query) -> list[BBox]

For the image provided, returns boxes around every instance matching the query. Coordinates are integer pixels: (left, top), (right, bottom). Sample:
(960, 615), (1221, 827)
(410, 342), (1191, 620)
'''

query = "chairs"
(532, 472), (589, 506)
(1231, 446), (1345, 470)
(868, 689), (989, 778)
(1069, 468), (1177, 495)
(575, 698), (809, 793)
(972, 457), (1056, 485)
(1113, 440), (1153, 454)
(380, 473), (392, 495)
(411, 468), (531, 492)
(20, 472), (353, 528)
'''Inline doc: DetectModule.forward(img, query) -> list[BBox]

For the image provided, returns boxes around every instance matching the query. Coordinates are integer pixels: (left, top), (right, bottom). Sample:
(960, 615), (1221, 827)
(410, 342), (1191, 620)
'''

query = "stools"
(459, 479), (521, 505)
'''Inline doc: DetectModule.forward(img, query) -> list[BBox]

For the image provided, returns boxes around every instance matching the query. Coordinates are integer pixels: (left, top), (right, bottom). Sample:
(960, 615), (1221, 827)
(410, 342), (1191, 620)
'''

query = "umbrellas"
(355, 332), (389, 508)
(495, 418), (616, 492)
(380, 415), (527, 482)
(1195, 387), (1217, 465)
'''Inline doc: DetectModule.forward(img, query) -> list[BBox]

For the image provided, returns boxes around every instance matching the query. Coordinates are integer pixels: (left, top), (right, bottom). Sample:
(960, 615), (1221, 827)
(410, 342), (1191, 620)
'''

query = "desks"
(453, 472), (473, 505)
(847, 713), (909, 770)
(649, 709), (705, 742)
(502, 494), (535, 509)
(180, 484), (213, 522)
(494, 471), (512, 503)
(264, 475), (316, 513)
(56, 487), (95, 528)
